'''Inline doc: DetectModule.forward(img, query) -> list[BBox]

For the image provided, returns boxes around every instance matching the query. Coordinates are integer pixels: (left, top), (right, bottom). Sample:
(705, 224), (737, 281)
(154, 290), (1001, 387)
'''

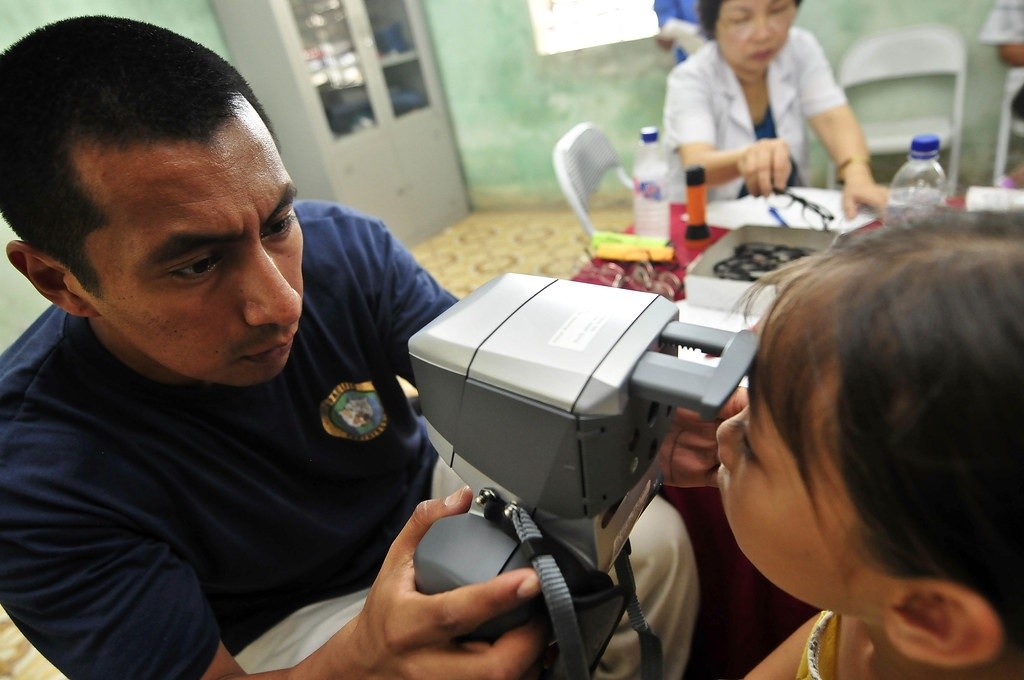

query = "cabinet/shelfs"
(209, 0), (472, 249)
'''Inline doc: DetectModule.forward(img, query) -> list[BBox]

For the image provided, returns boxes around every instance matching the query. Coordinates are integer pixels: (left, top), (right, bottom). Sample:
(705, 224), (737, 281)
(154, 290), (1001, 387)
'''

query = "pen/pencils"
(769, 207), (789, 227)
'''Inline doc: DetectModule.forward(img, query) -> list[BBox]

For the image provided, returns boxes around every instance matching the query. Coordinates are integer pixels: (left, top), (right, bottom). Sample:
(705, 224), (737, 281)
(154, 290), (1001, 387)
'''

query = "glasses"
(765, 187), (834, 232)
(713, 242), (815, 281)
(578, 248), (683, 302)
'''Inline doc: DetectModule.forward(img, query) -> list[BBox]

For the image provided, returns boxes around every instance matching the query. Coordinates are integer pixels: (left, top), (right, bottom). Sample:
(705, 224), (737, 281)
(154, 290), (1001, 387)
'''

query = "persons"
(714, 205), (1024, 680)
(981, 1), (1024, 186)
(0, 15), (748, 680)
(658, 0), (891, 224)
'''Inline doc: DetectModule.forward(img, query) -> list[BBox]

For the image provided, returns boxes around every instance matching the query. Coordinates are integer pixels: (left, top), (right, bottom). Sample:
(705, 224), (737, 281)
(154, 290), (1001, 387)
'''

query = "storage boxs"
(374, 20), (408, 54)
(684, 225), (842, 318)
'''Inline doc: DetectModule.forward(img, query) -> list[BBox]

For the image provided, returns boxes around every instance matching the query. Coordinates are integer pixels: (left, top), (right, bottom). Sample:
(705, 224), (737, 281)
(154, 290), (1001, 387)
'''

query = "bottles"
(630, 126), (670, 241)
(881, 135), (946, 231)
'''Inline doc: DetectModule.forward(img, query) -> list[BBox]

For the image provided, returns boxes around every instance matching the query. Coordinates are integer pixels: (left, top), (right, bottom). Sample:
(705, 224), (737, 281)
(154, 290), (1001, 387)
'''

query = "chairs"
(994, 65), (1024, 190)
(550, 123), (633, 236)
(826, 25), (967, 198)
